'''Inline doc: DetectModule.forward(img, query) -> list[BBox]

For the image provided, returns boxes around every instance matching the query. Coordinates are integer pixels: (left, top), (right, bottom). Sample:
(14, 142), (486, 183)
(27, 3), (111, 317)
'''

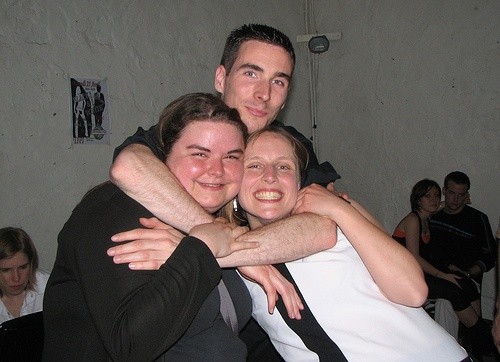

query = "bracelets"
(466, 269), (472, 277)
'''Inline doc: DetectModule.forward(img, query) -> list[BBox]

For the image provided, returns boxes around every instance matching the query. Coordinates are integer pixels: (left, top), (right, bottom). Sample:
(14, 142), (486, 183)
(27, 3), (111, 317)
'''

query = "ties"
(267, 262), (348, 362)
(219, 267), (253, 336)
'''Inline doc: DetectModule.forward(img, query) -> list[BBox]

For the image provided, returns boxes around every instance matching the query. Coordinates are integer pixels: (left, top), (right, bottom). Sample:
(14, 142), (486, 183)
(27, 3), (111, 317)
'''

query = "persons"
(391, 178), (500, 362)
(236, 128), (475, 362)
(425, 171), (496, 287)
(0, 227), (51, 327)
(44, 93), (285, 362)
(110, 23), (341, 362)
